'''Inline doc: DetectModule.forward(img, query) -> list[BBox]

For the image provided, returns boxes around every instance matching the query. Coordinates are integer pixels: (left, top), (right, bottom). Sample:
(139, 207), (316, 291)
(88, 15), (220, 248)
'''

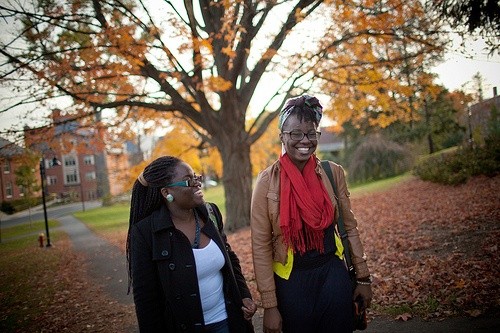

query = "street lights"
(39, 155), (61, 247)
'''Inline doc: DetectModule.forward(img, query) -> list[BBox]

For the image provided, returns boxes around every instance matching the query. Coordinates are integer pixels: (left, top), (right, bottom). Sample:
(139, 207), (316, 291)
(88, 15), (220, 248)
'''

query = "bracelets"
(357, 279), (372, 286)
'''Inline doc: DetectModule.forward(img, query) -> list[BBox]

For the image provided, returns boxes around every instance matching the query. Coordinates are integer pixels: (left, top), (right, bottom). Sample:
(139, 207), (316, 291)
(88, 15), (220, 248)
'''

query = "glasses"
(282, 128), (322, 141)
(165, 175), (204, 187)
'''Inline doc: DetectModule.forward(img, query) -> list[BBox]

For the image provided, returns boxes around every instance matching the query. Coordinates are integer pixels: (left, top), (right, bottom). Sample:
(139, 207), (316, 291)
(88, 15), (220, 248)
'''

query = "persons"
(250, 95), (374, 333)
(126, 155), (258, 333)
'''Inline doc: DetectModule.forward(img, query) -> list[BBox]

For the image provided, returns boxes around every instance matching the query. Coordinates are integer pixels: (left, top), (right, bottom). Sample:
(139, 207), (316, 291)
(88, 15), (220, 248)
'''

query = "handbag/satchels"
(351, 275), (368, 331)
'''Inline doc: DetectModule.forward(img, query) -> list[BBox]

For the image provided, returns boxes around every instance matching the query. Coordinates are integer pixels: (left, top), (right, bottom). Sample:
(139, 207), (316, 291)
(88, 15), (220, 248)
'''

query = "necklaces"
(192, 208), (202, 249)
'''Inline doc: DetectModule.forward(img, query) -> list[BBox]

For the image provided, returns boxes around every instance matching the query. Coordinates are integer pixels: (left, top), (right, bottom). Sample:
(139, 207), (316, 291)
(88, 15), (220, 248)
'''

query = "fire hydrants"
(38, 233), (46, 247)
